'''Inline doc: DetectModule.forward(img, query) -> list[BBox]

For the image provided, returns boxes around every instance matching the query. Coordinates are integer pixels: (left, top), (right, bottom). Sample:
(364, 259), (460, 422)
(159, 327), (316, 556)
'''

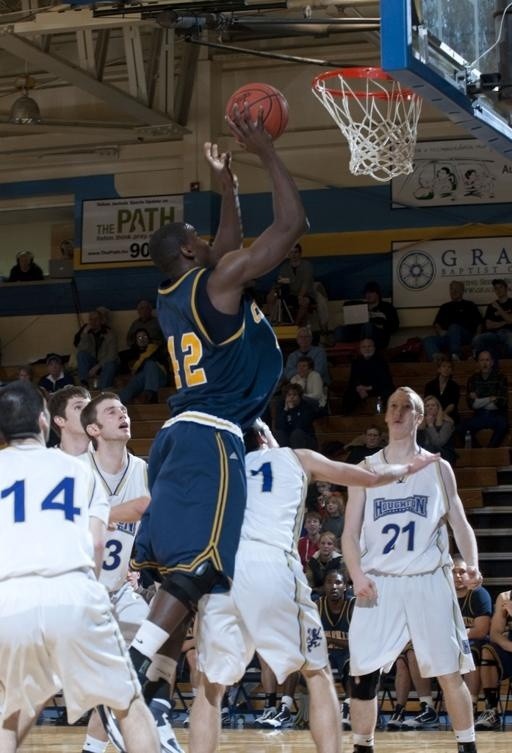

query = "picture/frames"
(387, 232), (511, 310)
(385, 136), (510, 212)
(77, 192), (183, 264)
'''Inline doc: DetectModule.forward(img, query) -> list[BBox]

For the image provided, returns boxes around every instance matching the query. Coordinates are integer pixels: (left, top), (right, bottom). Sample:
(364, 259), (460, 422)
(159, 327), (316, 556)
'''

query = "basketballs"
(227, 83), (289, 142)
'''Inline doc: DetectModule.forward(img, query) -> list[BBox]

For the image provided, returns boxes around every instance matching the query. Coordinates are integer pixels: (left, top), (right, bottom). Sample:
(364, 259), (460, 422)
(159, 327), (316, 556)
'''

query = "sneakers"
(96, 705), (126, 753)
(152, 709), (185, 753)
(221, 700), (501, 731)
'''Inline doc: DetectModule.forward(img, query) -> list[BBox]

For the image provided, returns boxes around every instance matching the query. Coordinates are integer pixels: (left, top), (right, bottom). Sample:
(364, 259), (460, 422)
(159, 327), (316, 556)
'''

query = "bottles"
(375, 396), (382, 414)
(464, 430), (471, 449)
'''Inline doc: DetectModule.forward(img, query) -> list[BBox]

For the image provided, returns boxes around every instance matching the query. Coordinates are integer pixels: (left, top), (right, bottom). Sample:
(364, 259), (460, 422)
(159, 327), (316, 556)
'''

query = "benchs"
(3, 317), (512, 733)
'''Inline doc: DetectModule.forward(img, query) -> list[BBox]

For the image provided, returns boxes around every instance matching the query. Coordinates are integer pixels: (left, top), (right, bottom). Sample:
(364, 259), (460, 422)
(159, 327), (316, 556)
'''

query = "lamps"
(9, 56), (39, 122)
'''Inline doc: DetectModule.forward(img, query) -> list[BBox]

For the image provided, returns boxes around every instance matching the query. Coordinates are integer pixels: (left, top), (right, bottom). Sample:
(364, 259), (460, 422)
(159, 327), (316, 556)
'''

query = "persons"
(0, 383), (440, 752)
(60, 239), (73, 260)
(242, 242), (512, 471)
(96, 100), (315, 753)
(340, 386), (483, 753)
(56, 481), (491, 728)
(474, 587), (511, 731)
(18, 299), (172, 405)
(9, 249), (45, 281)
